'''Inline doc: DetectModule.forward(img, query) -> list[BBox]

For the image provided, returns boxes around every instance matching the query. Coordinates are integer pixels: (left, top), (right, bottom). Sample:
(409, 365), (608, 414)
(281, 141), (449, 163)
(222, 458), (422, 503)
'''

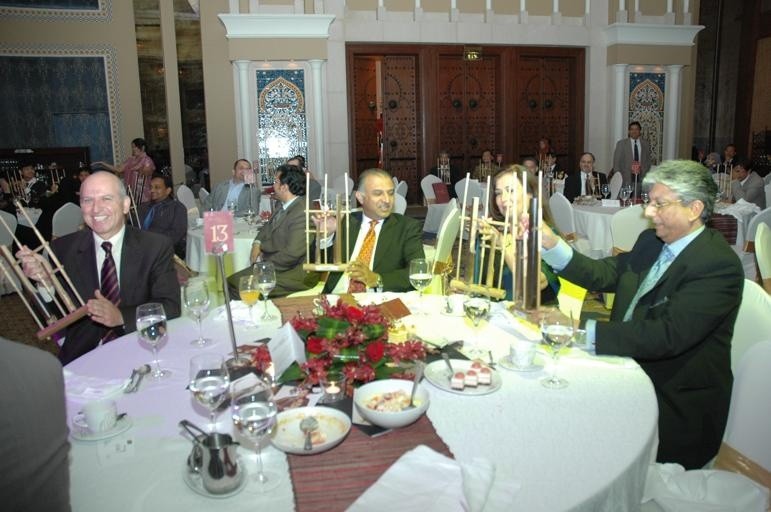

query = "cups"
(509, 341), (536, 368)
(73, 399), (119, 433)
(460, 456), (498, 511)
(196, 218), (205, 227)
(208, 200), (271, 228)
(318, 371), (347, 403)
(314, 294), (340, 311)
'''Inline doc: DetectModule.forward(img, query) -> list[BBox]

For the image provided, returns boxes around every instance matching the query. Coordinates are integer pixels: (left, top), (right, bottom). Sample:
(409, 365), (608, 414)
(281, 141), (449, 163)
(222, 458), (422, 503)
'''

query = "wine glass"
(183, 276), (218, 348)
(239, 260), (279, 329)
(540, 303), (575, 390)
(408, 257), (493, 330)
(232, 378), (283, 492)
(136, 302), (172, 381)
(590, 184), (650, 208)
(187, 353), (231, 424)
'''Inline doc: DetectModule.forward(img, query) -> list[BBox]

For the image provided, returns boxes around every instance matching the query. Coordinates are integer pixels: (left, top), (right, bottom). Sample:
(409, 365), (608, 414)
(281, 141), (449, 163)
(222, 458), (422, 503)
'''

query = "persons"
(518, 155), (743, 473)
(1, 337), (70, 512)
(611, 123), (653, 193)
(90, 138), (156, 206)
(17, 172), (183, 367)
(1, 141), (771, 299)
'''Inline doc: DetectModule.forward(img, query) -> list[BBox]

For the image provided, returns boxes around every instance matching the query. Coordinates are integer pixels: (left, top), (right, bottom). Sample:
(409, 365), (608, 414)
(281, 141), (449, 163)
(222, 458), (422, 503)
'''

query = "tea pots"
(179, 419), (250, 498)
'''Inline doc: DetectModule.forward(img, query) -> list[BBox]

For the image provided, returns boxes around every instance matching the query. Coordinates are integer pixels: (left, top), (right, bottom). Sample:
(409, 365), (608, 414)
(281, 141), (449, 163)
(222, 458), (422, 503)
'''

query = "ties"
(585, 174), (592, 196)
(271, 206), (284, 224)
(142, 206), (156, 231)
(622, 244), (675, 322)
(99, 242), (121, 345)
(634, 140), (639, 162)
(347, 218), (380, 294)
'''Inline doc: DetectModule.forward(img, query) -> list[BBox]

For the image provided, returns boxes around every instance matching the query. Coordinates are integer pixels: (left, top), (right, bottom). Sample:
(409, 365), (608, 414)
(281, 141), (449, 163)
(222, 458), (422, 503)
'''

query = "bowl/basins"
(356, 379), (433, 431)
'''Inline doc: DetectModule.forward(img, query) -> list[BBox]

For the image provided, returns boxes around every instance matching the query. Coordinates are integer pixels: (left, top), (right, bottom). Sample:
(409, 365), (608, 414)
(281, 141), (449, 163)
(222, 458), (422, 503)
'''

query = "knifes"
(125, 369), (136, 394)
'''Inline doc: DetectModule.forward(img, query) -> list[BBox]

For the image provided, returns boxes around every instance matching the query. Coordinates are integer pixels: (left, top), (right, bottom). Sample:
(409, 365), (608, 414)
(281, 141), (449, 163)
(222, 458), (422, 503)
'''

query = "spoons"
(405, 362), (426, 411)
(439, 351), (456, 380)
(130, 362), (153, 394)
(299, 414), (319, 451)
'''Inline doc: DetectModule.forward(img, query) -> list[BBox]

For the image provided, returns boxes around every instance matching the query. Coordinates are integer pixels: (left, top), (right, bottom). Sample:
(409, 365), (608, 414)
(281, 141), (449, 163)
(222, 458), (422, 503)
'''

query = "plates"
(502, 355), (544, 372)
(71, 413), (134, 443)
(312, 308), (325, 317)
(424, 359), (502, 396)
(270, 407), (353, 454)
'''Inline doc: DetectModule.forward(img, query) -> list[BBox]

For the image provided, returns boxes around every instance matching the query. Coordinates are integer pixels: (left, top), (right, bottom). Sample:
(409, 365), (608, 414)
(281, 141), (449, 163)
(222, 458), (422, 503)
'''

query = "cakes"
(451, 363), (492, 390)
